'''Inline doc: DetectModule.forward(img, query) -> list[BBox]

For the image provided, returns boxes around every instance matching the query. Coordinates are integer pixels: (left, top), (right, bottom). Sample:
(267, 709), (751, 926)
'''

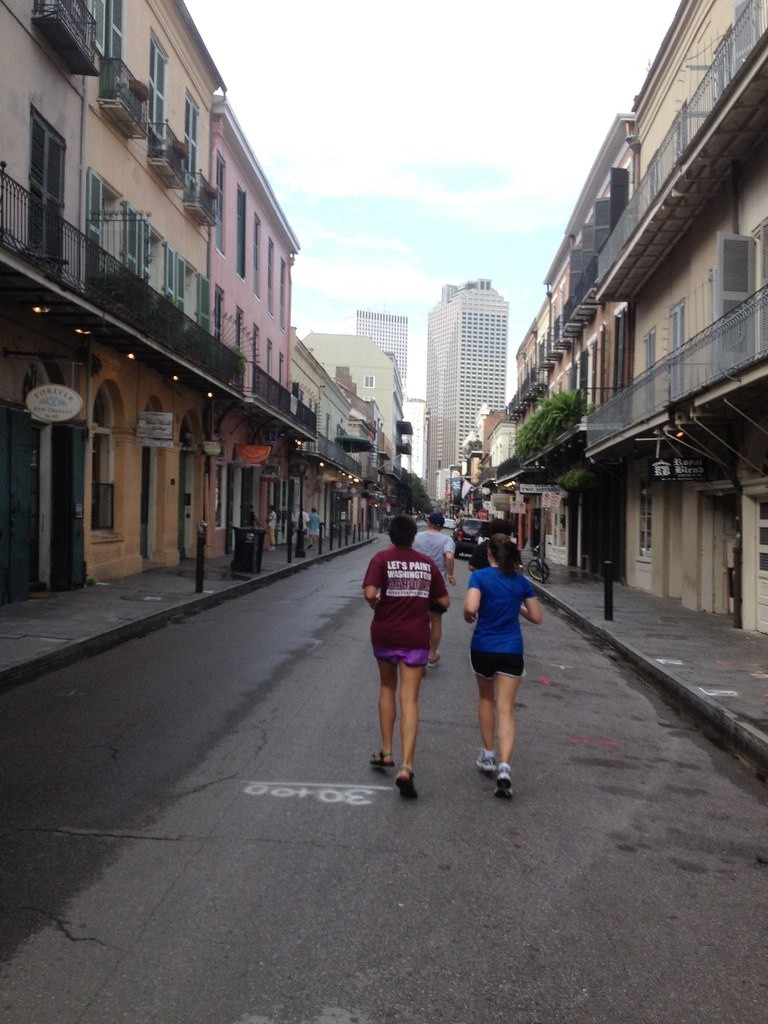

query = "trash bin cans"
(230, 525), (267, 574)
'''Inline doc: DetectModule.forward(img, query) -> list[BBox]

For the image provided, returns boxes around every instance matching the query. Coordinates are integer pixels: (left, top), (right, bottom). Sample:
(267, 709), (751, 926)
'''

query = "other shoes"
(266, 546), (275, 551)
(428, 650), (440, 666)
(307, 544), (313, 549)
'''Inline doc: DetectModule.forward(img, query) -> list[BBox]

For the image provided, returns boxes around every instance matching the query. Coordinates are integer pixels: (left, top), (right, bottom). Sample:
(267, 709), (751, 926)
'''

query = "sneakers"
(494, 767), (513, 799)
(475, 752), (496, 771)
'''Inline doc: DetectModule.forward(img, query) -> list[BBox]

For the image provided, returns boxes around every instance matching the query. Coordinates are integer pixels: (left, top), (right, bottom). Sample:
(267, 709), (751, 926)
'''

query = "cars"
(442, 519), (456, 530)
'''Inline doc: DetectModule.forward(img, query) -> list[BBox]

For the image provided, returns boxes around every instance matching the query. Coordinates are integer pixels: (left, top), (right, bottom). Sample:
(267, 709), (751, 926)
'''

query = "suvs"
(450, 516), (492, 560)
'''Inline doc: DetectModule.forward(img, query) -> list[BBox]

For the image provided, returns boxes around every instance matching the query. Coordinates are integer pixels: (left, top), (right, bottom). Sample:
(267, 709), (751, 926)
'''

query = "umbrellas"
(335, 433), (372, 460)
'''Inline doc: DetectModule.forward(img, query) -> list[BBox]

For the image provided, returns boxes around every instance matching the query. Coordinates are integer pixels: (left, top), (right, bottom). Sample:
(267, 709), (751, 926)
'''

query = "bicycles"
(527, 542), (552, 583)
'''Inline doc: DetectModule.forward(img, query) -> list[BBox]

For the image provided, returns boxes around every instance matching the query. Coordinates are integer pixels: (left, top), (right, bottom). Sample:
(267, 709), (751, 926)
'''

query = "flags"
(461, 480), (472, 499)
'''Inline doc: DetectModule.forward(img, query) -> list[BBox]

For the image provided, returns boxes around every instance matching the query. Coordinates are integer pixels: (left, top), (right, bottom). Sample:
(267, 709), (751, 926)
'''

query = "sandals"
(396, 766), (417, 798)
(370, 752), (395, 766)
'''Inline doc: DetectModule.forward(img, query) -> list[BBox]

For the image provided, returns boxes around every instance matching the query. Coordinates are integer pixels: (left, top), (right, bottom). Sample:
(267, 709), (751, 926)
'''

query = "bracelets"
(448, 576), (454, 579)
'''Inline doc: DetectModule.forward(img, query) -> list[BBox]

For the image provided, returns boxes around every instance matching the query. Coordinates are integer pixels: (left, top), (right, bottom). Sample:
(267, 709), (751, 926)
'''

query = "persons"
(411, 511), (456, 667)
(265, 505), (278, 551)
(468, 518), (523, 574)
(362, 514), (450, 799)
(308, 508), (320, 549)
(464, 534), (542, 799)
(250, 504), (257, 526)
(296, 506), (309, 541)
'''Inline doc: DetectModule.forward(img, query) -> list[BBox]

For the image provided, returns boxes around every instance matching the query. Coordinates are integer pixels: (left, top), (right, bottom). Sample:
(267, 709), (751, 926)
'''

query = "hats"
(427, 512), (444, 527)
